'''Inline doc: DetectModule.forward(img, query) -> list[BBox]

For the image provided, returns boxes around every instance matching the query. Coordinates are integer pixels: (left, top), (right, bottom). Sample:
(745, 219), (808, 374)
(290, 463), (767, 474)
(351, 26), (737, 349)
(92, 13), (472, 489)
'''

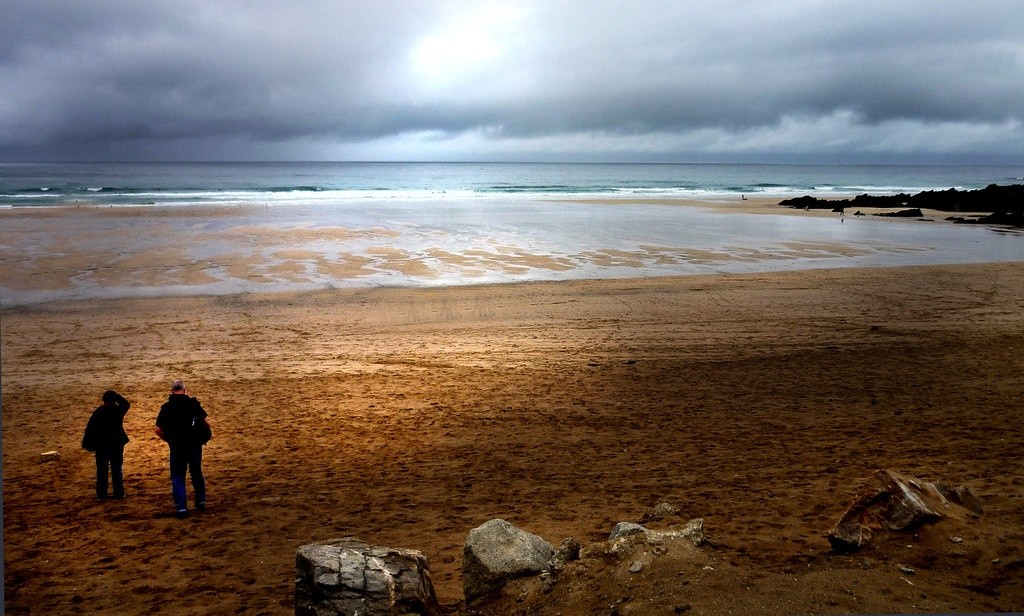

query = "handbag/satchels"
(188, 413), (211, 446)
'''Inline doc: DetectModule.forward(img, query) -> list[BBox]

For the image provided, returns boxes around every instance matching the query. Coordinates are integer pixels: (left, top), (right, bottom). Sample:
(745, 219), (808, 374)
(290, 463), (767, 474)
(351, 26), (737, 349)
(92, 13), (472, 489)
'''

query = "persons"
(83, 391), (130, 500)
(155, 380), (211, 511)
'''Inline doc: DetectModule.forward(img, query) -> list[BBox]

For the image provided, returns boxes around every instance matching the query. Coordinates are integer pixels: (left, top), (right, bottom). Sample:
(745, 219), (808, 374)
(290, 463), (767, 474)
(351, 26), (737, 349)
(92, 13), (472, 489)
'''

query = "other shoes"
(196, 503), (206, 512)
(176, 511), (188, 518)
